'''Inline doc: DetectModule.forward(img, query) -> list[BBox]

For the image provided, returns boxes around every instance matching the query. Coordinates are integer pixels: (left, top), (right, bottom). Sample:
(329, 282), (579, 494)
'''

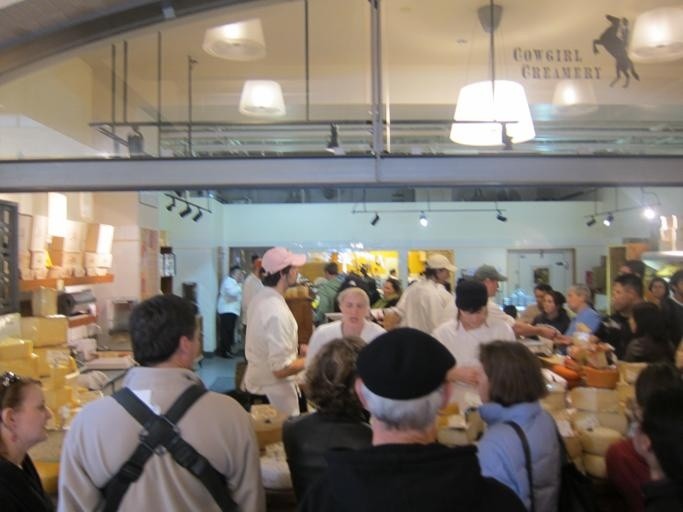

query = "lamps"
(496, 210), (506, 222)
(586, 218), (596, 227)
(166, 199), (176, 211)
(602, 216), (613, 227)
(370, 213), (380, 226)
(418, 212), (428, 227)
(202, 17), (268, 61)
(179, 204), (191, 218)
(324, 124), (341, 154)
(237, 78), (288, 117)
(446, 0), (539, 146)
(192, 210), (203, 222)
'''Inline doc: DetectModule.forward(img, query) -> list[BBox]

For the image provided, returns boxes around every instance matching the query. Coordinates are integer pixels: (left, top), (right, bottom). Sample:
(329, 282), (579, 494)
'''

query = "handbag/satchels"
(556, 421), (598, 512)
(334, 272), (376, 312)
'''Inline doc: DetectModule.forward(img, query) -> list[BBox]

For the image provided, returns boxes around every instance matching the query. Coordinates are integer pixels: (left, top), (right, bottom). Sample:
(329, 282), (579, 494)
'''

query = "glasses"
(632, 408), (645, 429)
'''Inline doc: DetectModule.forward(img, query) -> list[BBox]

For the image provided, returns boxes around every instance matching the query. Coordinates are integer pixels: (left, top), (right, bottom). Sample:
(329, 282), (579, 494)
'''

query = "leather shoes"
(223, 351), (232, 358)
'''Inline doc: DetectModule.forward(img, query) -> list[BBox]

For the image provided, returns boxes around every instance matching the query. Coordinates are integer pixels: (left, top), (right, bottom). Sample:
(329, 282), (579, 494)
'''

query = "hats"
(474, 263), (507, 282)
(548, 291), (566, 305)
(262, 247), (308, 276)
(455, 280), (488, 311)
(536, 283), (553, 292)
(356, 327), (456, 400)
(425, 253), (458, 273)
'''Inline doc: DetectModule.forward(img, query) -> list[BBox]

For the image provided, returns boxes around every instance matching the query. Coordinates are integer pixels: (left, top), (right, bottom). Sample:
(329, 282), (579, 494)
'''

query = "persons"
(58, 294), (266, 512)
(616, 259), (646, 279)
(387, 254), (459, 334)
(311, 262), (345, 327)
(239, 248), (309, 416)
(372, 278), (402, 308)
(333, 278), (373, 313)
(473, 265), (557, 340)
(632, 390), (683, 510)
(0, 370), (56, 512)
(475, 340), (562, 512)
(388, 269), (399, 281)
(296, 327), (526, 512)
(564, 285), (601, 337)
(527, 290), (571, 339)
(241, 258), (266, 346)
(281, 335), (367, 488)
(611, 275), (645, 359)
(502, 303), (517, 320)
(431, 279), (515, 405)
(625, 302), (674, 365)
(521, 284), (555, 324)
(602, 363), (679, 512)
(664, 271), (683, 367)
(648, 276), (669, 307)
(302, 287), (389, 369)
(215, 266), (243, 359)
